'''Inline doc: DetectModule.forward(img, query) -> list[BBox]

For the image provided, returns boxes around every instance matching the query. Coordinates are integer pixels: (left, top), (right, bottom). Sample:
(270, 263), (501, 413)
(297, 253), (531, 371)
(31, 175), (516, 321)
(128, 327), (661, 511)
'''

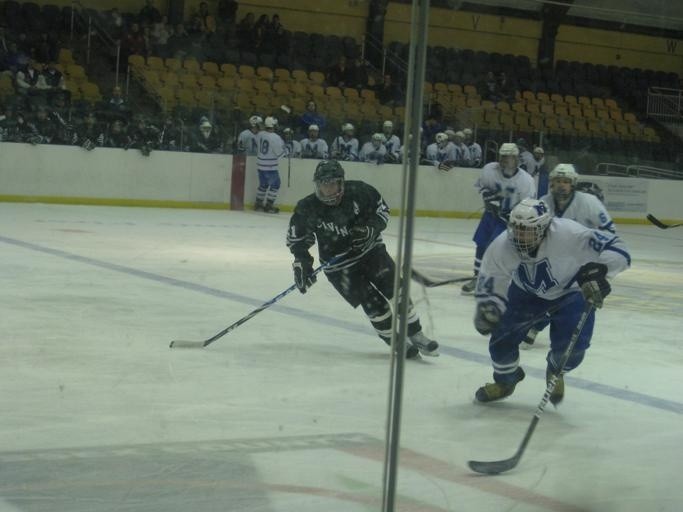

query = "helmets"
(549, 163), (579, 202)
(248, 116), (544, 160)
(312, 160), (345, 208)
(506, 197), (552, 256)
(497, 142), (520, 175)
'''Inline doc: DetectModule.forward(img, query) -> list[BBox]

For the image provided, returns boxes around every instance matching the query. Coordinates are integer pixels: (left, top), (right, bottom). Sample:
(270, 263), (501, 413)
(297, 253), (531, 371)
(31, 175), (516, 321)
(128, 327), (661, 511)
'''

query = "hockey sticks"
(468, 303), (594, 473)
(412, 269), (478, 288)
(647, 213), (683, 229)
(281, 104), (292, 187)
(170, 243), (353, 348)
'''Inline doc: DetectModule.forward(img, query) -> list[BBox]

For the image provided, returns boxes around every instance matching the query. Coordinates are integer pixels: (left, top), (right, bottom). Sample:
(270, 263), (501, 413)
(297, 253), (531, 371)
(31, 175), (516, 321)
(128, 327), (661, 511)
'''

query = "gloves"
(576, 269), (612, 309)
(384, 152), (397, 162)
(438, 163), (451, 172)
(346, 223), (377, 258)
(482, 192), (503, 217)
(291, 249), (317, 294)
(473, 300), (502, 335)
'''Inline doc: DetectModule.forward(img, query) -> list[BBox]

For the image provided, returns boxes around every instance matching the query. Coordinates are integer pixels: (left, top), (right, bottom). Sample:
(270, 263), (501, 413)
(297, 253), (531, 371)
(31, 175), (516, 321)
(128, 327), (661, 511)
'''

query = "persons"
(288, 161), (442, 361)
(1, 2), (408, 165)
(462, 142), (538, 293)
(524, 160), (619, 343)
(254, 117), (292, 214)
(420, 55), (552, 166)
(472, 195), (630, 408)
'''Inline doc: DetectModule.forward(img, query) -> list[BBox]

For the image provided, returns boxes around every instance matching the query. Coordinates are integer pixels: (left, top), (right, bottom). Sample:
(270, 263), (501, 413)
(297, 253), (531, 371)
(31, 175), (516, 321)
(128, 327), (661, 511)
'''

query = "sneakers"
(461, 280), (477, 292)
(264, 206), (280, 214)
(395, 343), (419, 358)
(254, 204), (265, 212)
(409, 331), (439, 352)
(475, 382), (515, 403)
(546, 363), (565, 405)
(523, 327), (538, 345)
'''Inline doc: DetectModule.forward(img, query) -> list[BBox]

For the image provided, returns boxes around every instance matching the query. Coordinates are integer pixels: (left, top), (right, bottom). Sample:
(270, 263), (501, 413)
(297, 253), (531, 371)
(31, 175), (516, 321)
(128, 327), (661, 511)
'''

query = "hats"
(199, 116), (212, 131)
